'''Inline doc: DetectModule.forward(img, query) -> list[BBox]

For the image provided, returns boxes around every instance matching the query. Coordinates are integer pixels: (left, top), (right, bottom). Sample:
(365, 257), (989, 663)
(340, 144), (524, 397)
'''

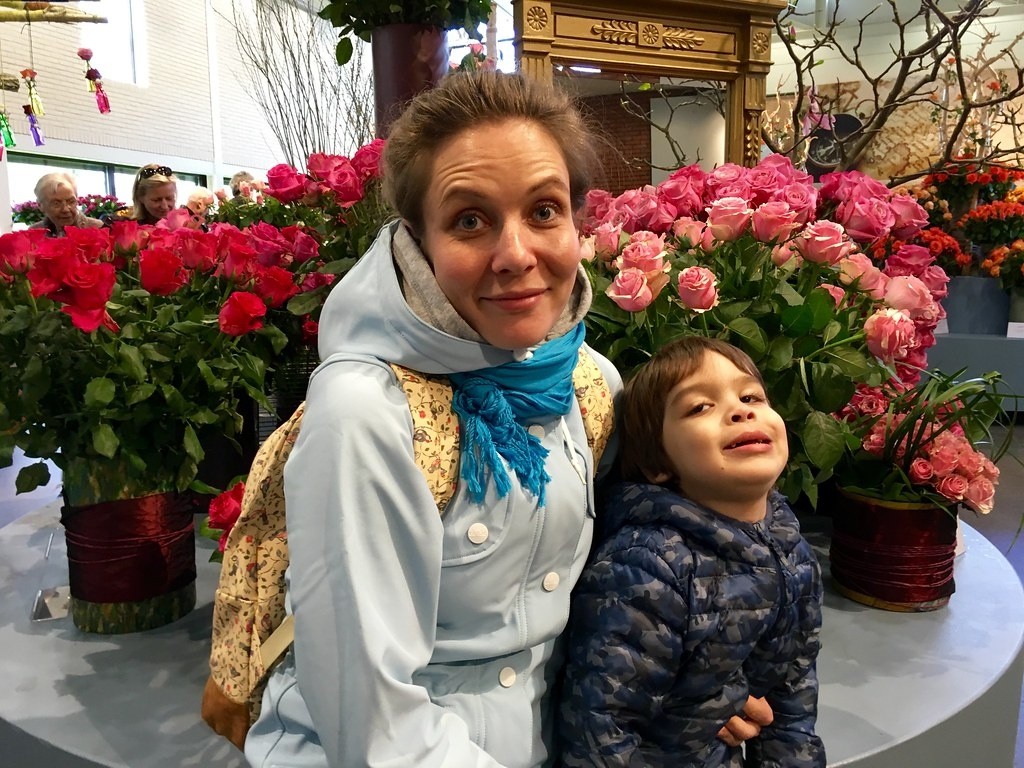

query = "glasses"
(136, 167), (172, 190)
(42, 199), (77, 209)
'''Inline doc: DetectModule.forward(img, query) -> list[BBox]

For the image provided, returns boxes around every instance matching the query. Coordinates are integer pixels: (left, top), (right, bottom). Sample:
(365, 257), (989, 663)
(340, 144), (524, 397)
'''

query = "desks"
(0, 491), (1024, 768)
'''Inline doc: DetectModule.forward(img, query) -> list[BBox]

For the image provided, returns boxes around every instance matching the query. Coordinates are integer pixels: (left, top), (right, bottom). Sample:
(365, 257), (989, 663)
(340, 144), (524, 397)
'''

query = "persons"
(243, 65), (623, 768)
(538, 338), (829, 768)
(131, 163), (259, 234)
(27, 170), (104, 238)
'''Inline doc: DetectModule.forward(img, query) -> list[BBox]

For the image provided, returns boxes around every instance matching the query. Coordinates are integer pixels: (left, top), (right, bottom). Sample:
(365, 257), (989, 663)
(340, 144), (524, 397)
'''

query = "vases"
(824, 488), (957, 613)
(943, 274), (1010, 336)
(49, 456), (195, 635)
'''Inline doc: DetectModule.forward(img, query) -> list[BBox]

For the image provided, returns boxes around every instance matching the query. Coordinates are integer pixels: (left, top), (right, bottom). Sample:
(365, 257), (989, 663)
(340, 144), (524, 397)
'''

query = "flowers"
(1, 149), (1023, 563)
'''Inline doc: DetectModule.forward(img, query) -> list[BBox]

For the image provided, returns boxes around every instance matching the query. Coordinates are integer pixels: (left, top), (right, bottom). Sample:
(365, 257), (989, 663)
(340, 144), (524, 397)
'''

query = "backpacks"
(201, 347), (615, 752)
